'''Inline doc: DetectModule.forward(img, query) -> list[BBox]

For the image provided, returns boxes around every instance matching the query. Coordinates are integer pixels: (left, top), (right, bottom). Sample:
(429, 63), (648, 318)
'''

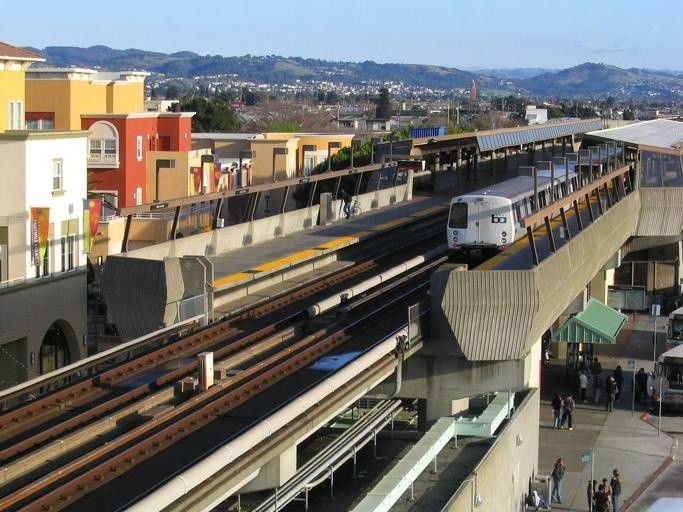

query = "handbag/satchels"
(346, 195), (352, 202)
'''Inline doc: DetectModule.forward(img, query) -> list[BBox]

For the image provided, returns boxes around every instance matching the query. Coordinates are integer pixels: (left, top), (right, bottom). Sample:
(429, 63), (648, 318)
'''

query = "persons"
(337, 188), (352, 220)
(543, 334), (551, 361)
(552, 458), (566, 504)
(466, 155), (473, 179)
(585, 469), (621, 512)
(612, 164), (634, 197)
(578, 355), (656, 412)
(550, 392), (575, 430)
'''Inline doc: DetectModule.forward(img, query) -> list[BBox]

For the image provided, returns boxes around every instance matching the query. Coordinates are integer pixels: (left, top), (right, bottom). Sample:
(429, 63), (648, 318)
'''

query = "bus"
(650, 343), (683, 412)
(664, 306), (683, 351)
(396, 158), (426, 173)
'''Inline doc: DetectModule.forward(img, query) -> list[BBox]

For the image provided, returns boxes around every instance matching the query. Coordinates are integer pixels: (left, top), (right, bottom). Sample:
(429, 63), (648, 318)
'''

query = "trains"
(446, 145), (635, 262)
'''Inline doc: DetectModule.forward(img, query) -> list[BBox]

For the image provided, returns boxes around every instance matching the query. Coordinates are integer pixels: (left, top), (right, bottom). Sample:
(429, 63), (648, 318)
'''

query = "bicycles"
(349, 200), (363, 219)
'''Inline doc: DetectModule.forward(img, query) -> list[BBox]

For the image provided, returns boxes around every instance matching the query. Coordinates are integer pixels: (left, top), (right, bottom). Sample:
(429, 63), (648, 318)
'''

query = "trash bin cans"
(530, 475), (551, 505)
(320, 192), (332, 226)
